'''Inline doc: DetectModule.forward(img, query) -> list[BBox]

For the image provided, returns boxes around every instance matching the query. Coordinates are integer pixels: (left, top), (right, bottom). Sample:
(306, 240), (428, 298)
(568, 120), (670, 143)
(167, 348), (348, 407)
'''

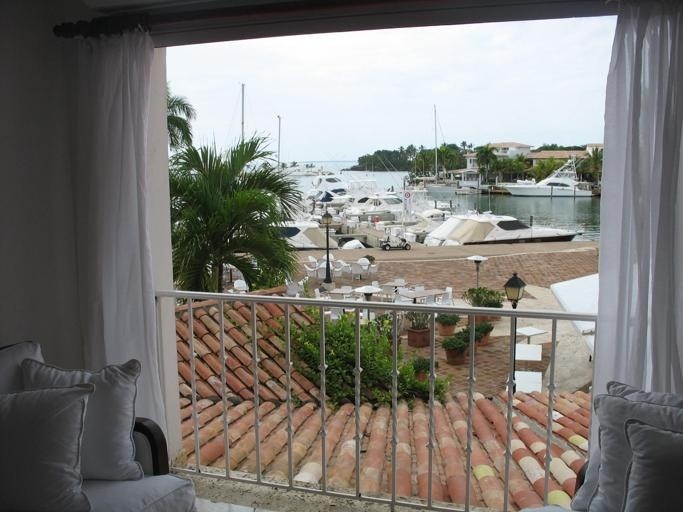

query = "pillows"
(586, 393), (683, 511)
(623, 418), (682, 512)
(15, 355), (145, 484)
(0, 385), (92, 512)
(571, 381), (683, 510)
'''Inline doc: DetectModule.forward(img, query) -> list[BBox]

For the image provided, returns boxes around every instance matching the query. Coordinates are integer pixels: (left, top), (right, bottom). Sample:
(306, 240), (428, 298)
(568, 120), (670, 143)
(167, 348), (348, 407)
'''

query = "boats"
(502, 177), (596, 197)
(504, 194), (592, 218)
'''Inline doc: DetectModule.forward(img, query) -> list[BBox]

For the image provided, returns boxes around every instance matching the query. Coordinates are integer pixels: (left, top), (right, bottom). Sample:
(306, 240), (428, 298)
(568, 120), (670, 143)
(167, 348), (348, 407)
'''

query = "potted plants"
(393, 286), (503, 363)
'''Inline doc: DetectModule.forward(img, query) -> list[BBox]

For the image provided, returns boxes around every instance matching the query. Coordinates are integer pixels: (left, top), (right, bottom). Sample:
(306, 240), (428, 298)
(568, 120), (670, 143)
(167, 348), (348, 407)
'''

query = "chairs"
(222, 252), (459, 307)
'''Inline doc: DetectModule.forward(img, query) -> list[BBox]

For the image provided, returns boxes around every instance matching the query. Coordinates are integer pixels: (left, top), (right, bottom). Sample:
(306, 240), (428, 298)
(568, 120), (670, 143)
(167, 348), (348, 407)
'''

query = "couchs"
(0, 333), (198, 512)
(564, 453), (589, 511)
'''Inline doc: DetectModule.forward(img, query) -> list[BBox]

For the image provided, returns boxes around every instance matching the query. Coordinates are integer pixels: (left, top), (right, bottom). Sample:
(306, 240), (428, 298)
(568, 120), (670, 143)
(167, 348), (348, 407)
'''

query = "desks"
(505, 328), (545, 394)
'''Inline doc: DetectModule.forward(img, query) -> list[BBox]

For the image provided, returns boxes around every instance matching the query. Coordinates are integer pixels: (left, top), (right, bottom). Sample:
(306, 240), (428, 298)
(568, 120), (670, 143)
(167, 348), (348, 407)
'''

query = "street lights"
(502, 272), (527, 393)
(321, 211), (332, 283)
